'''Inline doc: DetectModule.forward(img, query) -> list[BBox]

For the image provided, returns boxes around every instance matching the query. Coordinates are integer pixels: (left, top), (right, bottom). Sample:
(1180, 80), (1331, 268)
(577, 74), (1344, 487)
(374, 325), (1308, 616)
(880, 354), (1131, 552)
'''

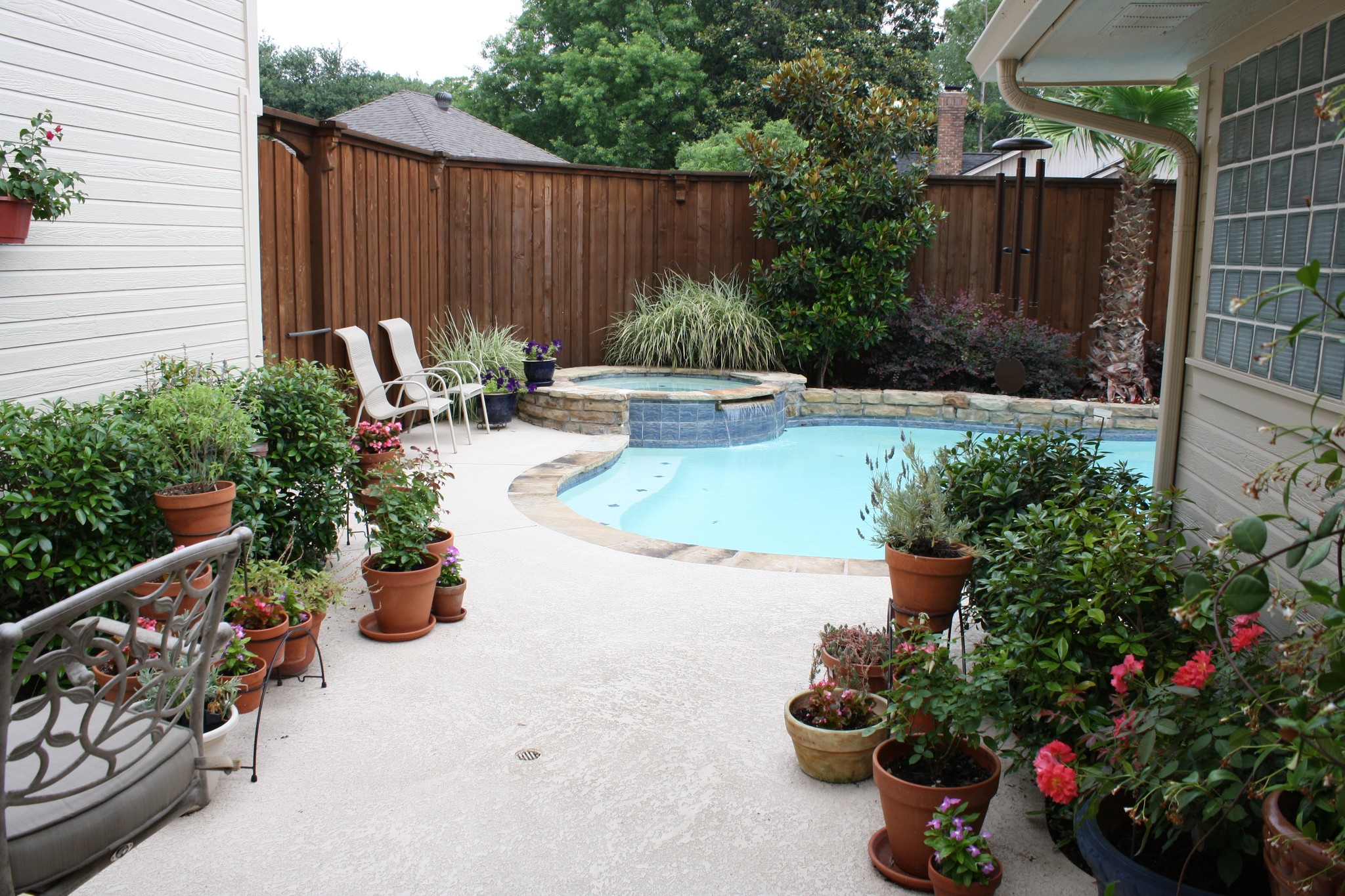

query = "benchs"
(1, 525), (250, 896)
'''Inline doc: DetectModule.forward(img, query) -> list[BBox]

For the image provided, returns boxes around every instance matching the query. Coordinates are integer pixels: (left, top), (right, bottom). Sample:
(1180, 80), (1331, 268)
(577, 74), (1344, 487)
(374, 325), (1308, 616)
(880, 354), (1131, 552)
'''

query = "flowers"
(0, 109), (88, 224)
(106, 422), (466, 727)
(477, 340), (565, 394)
(803, 262), (1345, 891)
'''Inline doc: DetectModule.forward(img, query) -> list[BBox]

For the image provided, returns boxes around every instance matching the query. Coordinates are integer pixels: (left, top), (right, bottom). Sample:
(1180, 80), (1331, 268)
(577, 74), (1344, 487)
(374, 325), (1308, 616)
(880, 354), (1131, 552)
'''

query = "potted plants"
(812, 623), (905, 698)
(1262, 783), (1345, 896)
(359, 445), (455, 631)
(1076, 776), (1269, 896)
(143, 652), (240, 763)
(857, 433), (975, 634)
(156, 381), (266, 535)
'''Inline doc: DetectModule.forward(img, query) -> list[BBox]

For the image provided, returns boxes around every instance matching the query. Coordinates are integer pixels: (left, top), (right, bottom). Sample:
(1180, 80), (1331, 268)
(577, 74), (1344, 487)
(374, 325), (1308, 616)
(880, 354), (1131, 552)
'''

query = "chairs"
(335, 318), (491, 468)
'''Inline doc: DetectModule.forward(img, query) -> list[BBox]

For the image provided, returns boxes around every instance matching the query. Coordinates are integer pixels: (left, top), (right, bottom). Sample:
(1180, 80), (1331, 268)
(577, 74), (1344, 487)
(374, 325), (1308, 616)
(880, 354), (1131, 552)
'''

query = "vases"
(232, 613), (288, 669)
(210, 656), (267, 714)
(353, 448), (405, 508)
(867, 733), (1002, 887)
(129, 560), (211, 619)
(888, 669), (954, 734)
(361, 485), (417, 524)
(928, 848), (1002, 896)
(475, 394), (518, 425)
(268, 585), (327, 679)
(400, 527), (454, 562)
(784, 689), (893, 785)
(521, 358), (557, 387)
(0, 196), (36, 244)
(431, 577), (466, 623)
(94, 650), (159, 709)
(280, 611), (312, 665)
